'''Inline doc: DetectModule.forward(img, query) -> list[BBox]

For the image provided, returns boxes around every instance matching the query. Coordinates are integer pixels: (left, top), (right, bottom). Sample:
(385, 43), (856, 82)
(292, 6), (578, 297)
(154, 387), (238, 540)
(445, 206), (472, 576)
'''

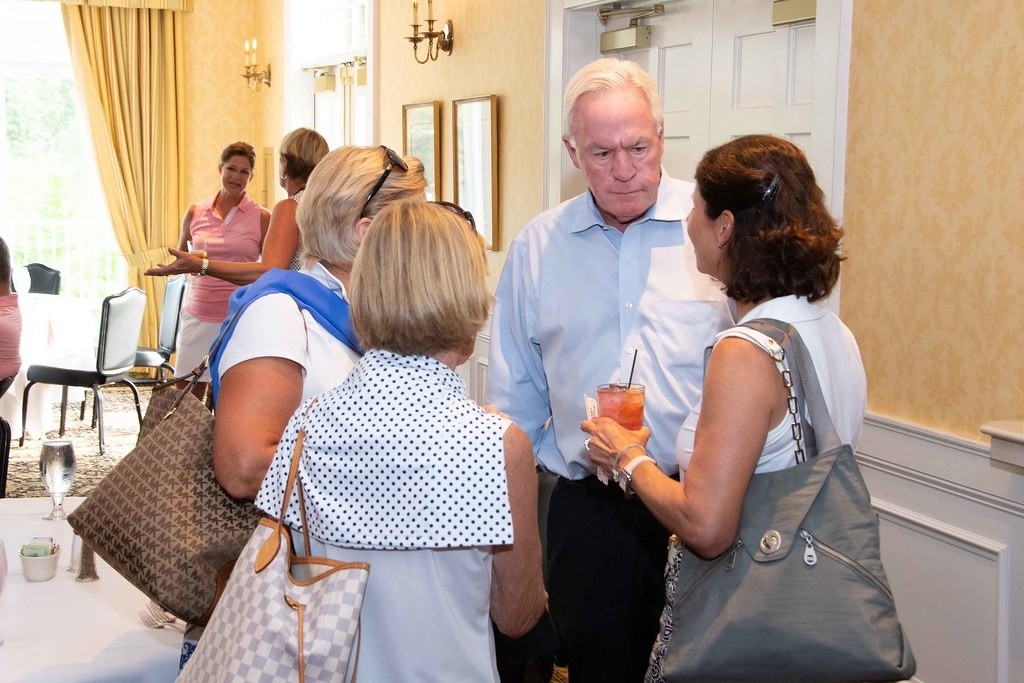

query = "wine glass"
(38, 440), (76, 521)
(0, 539), (8, 647)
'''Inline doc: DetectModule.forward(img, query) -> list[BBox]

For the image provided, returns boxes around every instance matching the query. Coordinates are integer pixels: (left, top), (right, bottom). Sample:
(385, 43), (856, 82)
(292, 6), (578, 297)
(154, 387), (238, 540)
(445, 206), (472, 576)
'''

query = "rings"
(584, 438), (592, 451)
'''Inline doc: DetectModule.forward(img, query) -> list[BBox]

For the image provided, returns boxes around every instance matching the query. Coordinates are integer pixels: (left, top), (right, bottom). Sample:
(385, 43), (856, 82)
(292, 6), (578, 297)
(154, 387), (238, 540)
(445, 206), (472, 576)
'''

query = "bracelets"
(197, 258), (209, 278)
(613, 445), (646, 483)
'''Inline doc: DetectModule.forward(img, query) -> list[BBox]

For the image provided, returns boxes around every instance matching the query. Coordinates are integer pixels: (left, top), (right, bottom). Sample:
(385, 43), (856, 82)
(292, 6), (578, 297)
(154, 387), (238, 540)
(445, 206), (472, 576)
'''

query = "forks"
(136, 601), (185, 634)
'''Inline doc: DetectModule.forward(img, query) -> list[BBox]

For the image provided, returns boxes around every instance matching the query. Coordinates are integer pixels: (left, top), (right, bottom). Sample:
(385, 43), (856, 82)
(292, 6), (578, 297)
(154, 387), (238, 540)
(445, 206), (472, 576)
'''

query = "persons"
(0, 238), (22, 383)
(144, 126), (330, 285)
(579, 134), (869, 683)
(253, 200), (547, 683)
(173, 142), (272, 403)
(484, 54), (740, 683)
(179, 145), (428, 676)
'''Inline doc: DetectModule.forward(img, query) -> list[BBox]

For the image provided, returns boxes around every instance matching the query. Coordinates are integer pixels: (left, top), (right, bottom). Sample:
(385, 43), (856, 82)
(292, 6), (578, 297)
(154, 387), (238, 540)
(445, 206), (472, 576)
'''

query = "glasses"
(360, 144), (408, 219)
(427, 201), (477, 237)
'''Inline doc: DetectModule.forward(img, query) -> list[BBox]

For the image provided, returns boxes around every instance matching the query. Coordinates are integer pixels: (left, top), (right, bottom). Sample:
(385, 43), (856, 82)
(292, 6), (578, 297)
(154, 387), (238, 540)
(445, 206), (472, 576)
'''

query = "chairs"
(20, 286), (148, 452)
(10, 263), (61, 294)
(80, 272), (190, 428)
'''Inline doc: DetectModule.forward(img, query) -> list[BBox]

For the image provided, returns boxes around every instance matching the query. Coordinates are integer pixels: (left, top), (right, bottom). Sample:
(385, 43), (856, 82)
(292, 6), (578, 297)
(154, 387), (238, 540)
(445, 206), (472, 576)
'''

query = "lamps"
(241, 38), (271, 92)
(404, 0), (454, 63)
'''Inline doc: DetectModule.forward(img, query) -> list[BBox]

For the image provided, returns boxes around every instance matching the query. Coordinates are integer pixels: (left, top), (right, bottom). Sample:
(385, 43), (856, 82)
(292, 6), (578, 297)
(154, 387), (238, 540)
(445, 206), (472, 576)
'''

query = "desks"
(0, 293), (107, 439)
(0, 497), (186, 682)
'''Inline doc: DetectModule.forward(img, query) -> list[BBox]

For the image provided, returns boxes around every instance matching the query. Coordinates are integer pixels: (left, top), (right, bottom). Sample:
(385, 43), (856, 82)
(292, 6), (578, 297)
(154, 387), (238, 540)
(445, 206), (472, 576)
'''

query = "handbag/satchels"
(175, 397), (369, 683)
(66, 288), (309, 628)
(643, 316), (916, 683)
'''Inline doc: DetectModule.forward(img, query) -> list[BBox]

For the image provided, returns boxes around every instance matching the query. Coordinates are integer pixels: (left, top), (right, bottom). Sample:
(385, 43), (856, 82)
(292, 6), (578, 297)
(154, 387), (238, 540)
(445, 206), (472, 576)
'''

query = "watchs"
(619, 456), (657, 496)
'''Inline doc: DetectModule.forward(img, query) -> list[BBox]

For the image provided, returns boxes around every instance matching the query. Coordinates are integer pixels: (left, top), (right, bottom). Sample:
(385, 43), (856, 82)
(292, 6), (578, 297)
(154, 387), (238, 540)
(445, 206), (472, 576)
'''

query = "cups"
(187, 240), (207, 276)
(18, 544), (62, 583)
(596, 383), (646, 430)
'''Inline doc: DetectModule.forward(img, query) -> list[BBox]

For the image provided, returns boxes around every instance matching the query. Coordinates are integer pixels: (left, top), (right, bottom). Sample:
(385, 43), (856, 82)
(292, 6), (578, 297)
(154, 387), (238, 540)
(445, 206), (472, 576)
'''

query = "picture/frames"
(401, 100), (441, 201)
(451, 94), (498, 251)
